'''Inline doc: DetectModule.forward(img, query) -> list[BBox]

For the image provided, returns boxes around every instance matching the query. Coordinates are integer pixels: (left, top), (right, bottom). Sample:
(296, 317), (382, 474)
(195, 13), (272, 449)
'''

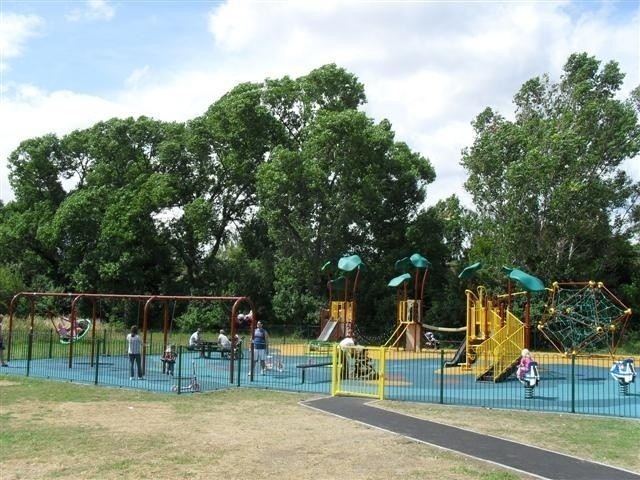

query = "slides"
(316, 316), (340, 342)
(443, 336), (466, 367)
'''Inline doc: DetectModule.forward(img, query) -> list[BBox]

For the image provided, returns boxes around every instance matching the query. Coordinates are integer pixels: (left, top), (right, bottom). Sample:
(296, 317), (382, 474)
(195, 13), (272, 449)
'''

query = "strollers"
(351, 353), (379, 381)
(423, 332), (441, 350)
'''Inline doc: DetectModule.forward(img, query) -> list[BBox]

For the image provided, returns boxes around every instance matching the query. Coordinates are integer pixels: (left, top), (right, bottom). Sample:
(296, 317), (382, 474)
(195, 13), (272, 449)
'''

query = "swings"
(223, 299), (257, 325)
(99, 298), (115, 358)
(160, 300), (190, 365)
(36, 296), (91, 343)
(122, 298), (142, 355)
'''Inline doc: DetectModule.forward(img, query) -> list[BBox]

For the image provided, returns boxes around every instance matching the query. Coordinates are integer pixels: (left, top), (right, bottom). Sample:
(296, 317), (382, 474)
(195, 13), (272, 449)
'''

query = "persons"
(425, 329), (433, 339)
(237, 310), (254, 330)
(58, 323), (70, 340)
(0, 314), (8, 367)
(336, 335), (369, 380)
(217, 329), (230, 351)
(166, 344), (177, 376)
(247, 320), (269, 376)
(515, 348), (534, 381)
(189, 328), (206, 359)
(127, 325), (149, 380)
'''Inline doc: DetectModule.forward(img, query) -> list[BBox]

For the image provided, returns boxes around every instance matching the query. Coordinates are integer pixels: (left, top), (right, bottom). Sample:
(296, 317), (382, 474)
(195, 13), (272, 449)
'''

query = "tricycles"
(260, 352), (285, 374)
